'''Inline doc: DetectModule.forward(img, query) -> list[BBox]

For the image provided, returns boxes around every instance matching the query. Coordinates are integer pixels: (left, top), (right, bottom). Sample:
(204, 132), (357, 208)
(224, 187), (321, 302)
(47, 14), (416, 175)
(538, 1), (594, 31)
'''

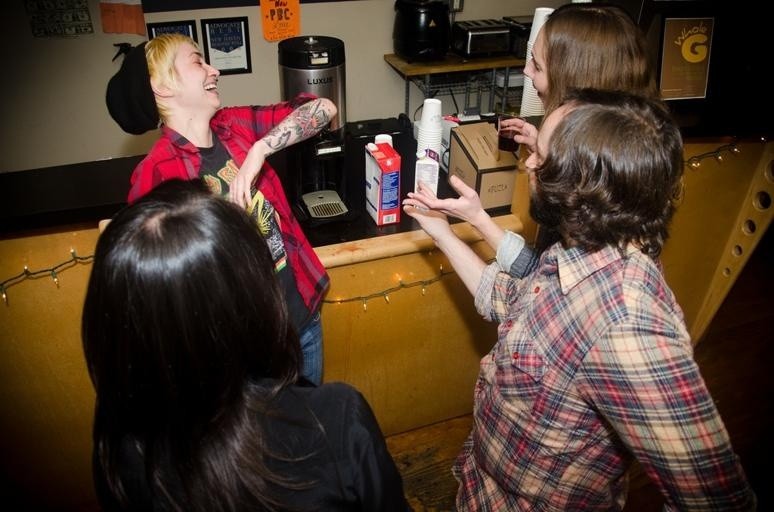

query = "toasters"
(454, 17), (513, 57)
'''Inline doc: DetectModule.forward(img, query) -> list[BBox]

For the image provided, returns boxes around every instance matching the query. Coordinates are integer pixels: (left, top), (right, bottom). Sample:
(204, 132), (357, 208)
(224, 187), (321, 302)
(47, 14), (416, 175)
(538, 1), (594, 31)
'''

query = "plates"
(413, 120), (459, 144)
(488, 68), (524, 87)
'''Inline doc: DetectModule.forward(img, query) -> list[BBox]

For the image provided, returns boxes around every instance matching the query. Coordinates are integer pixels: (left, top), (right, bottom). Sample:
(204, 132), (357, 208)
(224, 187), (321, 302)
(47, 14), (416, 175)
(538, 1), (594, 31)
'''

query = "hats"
(106, 41), (158, 135)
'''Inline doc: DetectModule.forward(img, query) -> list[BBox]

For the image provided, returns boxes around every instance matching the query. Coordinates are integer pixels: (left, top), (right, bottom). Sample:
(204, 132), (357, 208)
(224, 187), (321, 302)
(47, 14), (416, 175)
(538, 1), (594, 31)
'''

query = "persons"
(403, 93), (760, 510)
(405, 4), (656, 283)
(103, 32), (338, 389)
(81, 178), (409, 511)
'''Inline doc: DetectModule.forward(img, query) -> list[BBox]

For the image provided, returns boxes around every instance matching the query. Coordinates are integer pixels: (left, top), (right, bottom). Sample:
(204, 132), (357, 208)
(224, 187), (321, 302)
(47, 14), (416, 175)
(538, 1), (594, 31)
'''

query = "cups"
(374, 133), (393, 149)
(517, 5), (556, 118)
(497, 114), (526, 153)
(416, 97), (443, 161)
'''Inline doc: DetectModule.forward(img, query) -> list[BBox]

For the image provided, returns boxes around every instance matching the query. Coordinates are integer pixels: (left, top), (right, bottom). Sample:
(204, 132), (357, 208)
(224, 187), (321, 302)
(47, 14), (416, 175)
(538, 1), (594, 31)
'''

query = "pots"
(393, 0), (464, 64)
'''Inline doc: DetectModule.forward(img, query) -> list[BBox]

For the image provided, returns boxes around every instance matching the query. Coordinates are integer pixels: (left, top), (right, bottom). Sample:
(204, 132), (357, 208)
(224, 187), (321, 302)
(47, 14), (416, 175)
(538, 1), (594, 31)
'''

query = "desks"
(384, 52), (527, 122)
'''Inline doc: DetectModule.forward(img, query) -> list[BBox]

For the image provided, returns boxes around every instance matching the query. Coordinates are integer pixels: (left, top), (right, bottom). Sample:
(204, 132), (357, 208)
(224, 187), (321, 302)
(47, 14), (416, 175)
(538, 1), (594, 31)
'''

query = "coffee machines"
(276, 35), (360, 234)
